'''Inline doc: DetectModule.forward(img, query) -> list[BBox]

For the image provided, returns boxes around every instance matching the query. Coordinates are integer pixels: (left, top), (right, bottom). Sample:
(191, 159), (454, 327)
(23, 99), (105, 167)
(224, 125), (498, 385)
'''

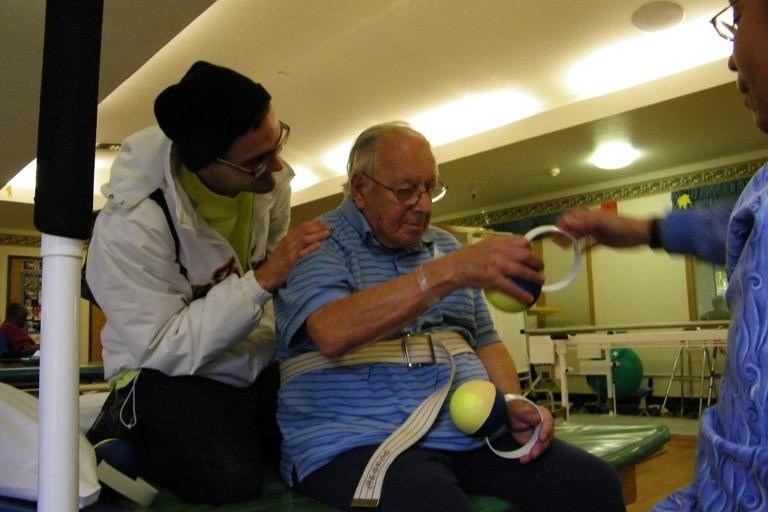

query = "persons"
(1, 300), (40, 358)
(81, 57), (332, 512)
(551, 0), (768, 511)
(272, 120), (627, 511)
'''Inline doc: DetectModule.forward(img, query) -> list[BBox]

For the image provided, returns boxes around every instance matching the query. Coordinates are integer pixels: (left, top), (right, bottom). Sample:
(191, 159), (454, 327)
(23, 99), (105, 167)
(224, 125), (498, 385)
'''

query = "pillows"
(0, 380), (101, 510)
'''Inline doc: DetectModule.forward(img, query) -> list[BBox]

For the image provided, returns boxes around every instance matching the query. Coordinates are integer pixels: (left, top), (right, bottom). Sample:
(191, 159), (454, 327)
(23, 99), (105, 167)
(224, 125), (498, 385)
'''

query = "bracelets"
(648, 216), (662, 252)
(414, 261), (442, 310)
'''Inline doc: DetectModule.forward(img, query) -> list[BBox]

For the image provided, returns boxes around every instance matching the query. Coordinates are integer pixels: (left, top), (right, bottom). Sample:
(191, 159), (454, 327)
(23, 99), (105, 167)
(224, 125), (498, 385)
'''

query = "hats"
(153, 60), (272, 173)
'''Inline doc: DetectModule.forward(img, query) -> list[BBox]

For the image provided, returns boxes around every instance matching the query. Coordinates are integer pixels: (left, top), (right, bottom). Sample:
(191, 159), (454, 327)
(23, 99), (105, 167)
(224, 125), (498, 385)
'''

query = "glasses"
(215, 118), (292, 180)
(360, 171), (449, 207)
(710, 0), (741, 41)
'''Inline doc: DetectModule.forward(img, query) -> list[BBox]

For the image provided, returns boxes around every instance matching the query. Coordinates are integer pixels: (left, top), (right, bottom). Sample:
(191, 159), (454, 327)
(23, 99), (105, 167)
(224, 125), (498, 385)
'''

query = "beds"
(2, 425), (671, 512)
